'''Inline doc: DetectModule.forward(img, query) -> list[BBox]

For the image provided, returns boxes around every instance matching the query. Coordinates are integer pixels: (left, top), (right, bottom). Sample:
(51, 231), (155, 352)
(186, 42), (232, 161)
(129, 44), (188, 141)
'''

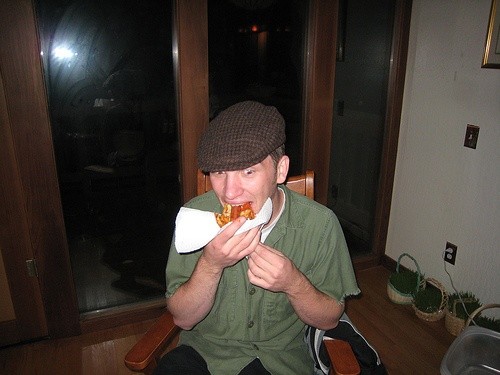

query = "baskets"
(445, 309), (465, 336)
(387, 253), (427, 304)
(412, 277), (449, 322)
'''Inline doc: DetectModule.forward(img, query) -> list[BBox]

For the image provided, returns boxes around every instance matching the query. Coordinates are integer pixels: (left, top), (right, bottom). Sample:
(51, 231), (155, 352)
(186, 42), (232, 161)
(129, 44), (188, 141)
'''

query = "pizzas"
(215, 203), (256, 229)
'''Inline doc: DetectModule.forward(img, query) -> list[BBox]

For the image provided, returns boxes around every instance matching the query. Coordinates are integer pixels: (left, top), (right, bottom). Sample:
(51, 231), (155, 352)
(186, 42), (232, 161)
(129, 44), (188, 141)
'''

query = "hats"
(198, 101), (286, 172)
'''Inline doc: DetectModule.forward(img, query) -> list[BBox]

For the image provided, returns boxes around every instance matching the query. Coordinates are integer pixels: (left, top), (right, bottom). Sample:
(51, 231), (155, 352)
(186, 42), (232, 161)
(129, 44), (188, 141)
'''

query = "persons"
(153, 101), (361, 375)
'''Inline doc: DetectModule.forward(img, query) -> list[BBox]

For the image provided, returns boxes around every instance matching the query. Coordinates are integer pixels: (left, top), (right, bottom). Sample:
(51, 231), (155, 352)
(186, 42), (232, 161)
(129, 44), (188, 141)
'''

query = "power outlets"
(445, 241), (458, 264)
(463, 124), (480, 149)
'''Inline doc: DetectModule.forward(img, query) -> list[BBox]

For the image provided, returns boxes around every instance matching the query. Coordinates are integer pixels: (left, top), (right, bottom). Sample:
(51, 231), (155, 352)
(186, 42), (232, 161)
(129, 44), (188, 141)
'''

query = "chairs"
(123, 168), (363, 375)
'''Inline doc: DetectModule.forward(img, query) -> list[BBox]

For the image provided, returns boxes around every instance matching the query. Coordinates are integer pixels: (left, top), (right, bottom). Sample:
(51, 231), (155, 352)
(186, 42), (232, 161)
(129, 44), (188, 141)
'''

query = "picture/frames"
(480, 0), (500, 71)
